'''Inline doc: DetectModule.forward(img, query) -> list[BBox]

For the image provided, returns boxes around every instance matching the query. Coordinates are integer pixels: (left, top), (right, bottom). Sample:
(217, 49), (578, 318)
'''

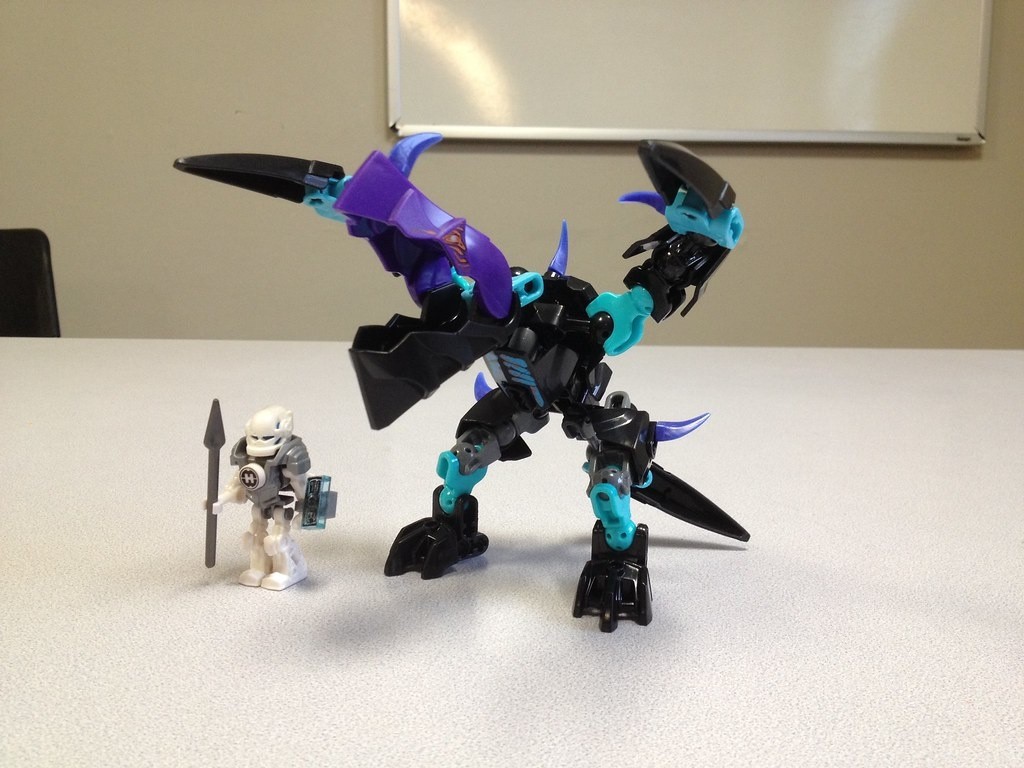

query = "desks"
(0, 337), (1024, 768)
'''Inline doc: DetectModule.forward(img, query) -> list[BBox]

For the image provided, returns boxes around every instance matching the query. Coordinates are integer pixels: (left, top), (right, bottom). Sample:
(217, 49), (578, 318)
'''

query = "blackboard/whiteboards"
(385, 0), (994, 147)
